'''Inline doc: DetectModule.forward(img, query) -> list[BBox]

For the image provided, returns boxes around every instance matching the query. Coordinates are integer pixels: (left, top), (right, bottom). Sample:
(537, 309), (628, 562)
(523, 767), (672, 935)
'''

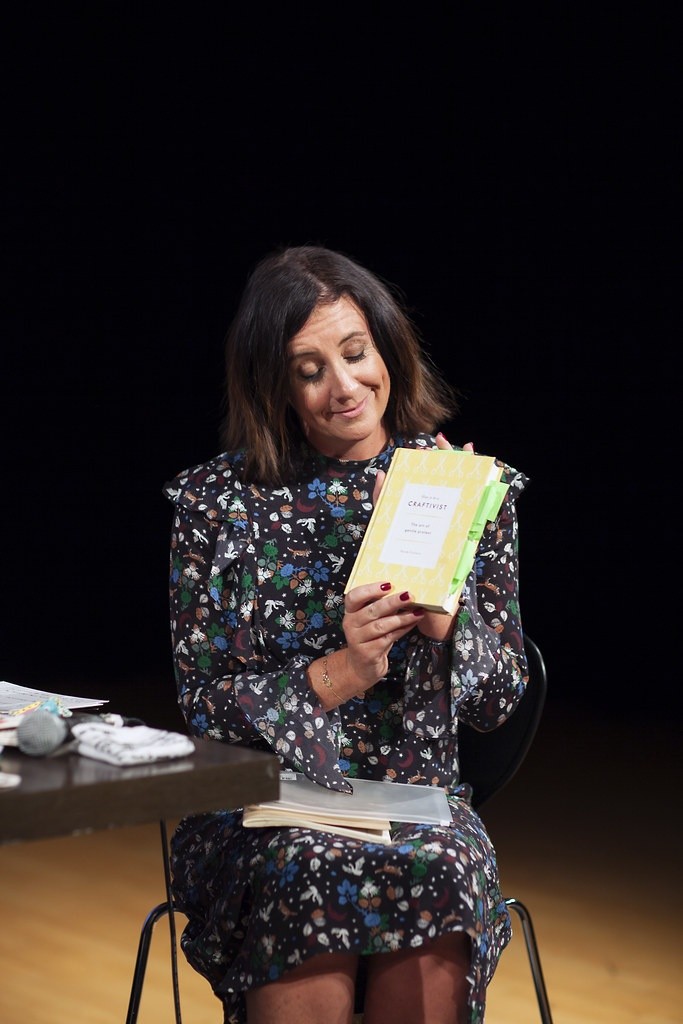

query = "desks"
(0, 733), (281, 842)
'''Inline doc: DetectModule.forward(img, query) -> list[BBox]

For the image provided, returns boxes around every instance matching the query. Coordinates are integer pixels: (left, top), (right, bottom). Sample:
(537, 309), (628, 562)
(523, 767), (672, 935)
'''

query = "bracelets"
(321, 657), (346, 703)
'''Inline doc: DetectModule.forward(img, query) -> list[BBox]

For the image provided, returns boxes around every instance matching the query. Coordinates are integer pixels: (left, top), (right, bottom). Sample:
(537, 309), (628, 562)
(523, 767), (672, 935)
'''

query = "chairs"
(125, 630), (554, 1024)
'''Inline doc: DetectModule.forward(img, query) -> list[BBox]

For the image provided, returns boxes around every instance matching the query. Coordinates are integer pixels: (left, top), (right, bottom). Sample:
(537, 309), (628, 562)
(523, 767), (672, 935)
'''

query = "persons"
(161, 248), (531, 1024)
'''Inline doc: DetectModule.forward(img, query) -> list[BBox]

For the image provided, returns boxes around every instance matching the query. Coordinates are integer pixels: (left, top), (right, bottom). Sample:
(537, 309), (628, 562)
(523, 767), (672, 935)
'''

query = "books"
(243, 803), (392, 845)
(344, 448), (504, 616)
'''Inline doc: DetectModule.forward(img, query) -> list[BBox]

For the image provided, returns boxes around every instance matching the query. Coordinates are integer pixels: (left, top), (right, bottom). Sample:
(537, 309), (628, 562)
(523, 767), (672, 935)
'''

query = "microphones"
(16, 710), (132, 755)
